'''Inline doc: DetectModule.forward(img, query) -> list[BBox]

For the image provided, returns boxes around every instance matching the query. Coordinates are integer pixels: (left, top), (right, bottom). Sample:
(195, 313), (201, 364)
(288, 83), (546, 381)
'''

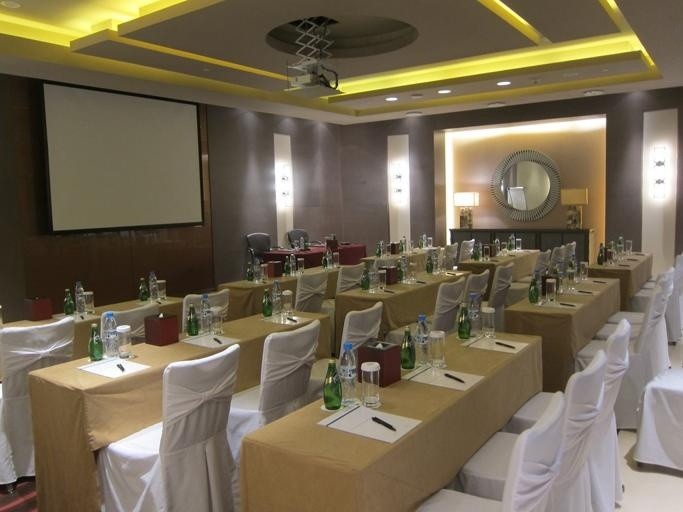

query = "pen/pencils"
(560, 303), (576, 307)
(578, 291), (593, 294)
(619, 265), (630, 267)
(372, 417), (396, 431)
(636, 254), (644, 255)
(416, 281), (426, 284)
(445, 374), (465, 383)
(117, 364), (124, 371)
(592, 281), (607, 284)
(495, 342), (515, 349)
(214, 338), (221, 344)
(628, 259), (638, 261)
(380, 287), (394, 293)
(447, 273), (456, 275)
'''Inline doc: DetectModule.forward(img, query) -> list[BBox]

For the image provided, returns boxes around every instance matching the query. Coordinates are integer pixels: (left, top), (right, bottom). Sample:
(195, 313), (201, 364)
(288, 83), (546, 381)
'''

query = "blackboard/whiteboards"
(40, 83), (204, 235)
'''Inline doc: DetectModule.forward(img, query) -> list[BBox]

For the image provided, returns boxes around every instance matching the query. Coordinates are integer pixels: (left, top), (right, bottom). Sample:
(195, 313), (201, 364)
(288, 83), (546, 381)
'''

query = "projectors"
(291, 71), (329, 87)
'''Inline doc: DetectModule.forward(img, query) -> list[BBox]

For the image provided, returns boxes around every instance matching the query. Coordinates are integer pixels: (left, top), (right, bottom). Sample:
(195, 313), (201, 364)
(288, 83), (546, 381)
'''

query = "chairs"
(575, 286), (663, 432)
(243, 221), (683, 305)
(181, 289), (231, 332)
(417, 390), (565, 510)
(501, 318), (631, 510)
(0, 316), (76, 497)
(631, 367), (683, 473)
(631, 263), (682, 346)
(99, 301), (160, 344)
(384, 276), (465, 346)
(593, 275), (674, 370)
(227, 317), (321, 458)
(305, 301), (383, 403)
(458, 351), (606, 510)
(507, 186), (526, 211)
(95, 343), (240, 510)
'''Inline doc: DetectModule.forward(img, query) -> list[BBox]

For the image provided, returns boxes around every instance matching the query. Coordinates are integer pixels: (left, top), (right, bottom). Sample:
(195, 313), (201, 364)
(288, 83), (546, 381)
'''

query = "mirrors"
(489, 148), (562, 224)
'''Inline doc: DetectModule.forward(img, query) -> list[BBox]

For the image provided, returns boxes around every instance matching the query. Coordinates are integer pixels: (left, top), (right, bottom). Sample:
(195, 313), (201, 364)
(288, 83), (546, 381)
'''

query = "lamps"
(650, 178), (666, 201)
(454, 191), (479, 229)
(651, 144), (666, 166)
(559, 187), (590, 229)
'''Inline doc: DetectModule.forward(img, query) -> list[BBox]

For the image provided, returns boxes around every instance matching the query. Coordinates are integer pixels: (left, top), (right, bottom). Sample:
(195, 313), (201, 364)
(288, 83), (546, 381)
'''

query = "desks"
(239, 330), (543, 510)
(504, 275), (620, 394)
(28, 310), (333, 510)
(334, 270), (472, 359)
(217, 264), (343, 320)
(0, 296), (185, 359)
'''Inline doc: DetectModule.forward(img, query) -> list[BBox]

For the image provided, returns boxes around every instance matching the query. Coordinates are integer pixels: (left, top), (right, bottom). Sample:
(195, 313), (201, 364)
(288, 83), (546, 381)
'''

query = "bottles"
(116, 323), (135, 360)
(597, 236), (645, 267)
(187, 294), (222, 337)
(414, 313), (432, 366)
(361, 234), (445, 292)
(470, 234), (521, 262)
(261, 280), (293, 317)
(299, 236), (305, 250)
(323, 360), (343, 410)
(399, 325), (415, 369)
(88, 323), (104, 361)
(101, 311), (118, 359)
(321, 246), (339, 270)
(138, 269), (166, 301)
(284, 253), (304, 277)
(246, 257), (269, 283)
(429, 330), (445, 368)
(467, 293), (483, 338)
(360, 361), (380, 409)
(64, 278), (95, 315)
(481, 306), (496, 341)
(339, 342), (358, 407)
(527, 258), (588, 304)
(457, 302), (470, 339)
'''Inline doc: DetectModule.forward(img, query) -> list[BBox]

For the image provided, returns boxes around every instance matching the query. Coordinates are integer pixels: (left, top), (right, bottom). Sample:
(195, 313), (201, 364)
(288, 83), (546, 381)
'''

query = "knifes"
(592, 280), (608, 284)
(578, 290), (592, 294)
(444, 374), (465, 384)
(559, 302), (575, 307)
(155, 300), (161, 304)
(495, 342), (516, 351)
(116, 363), (125, 372)
(213, 337), (222, 344)
(446, 272), (455, 276)
(369, 416), (396, 432)
(80, 315), (83, 319)
